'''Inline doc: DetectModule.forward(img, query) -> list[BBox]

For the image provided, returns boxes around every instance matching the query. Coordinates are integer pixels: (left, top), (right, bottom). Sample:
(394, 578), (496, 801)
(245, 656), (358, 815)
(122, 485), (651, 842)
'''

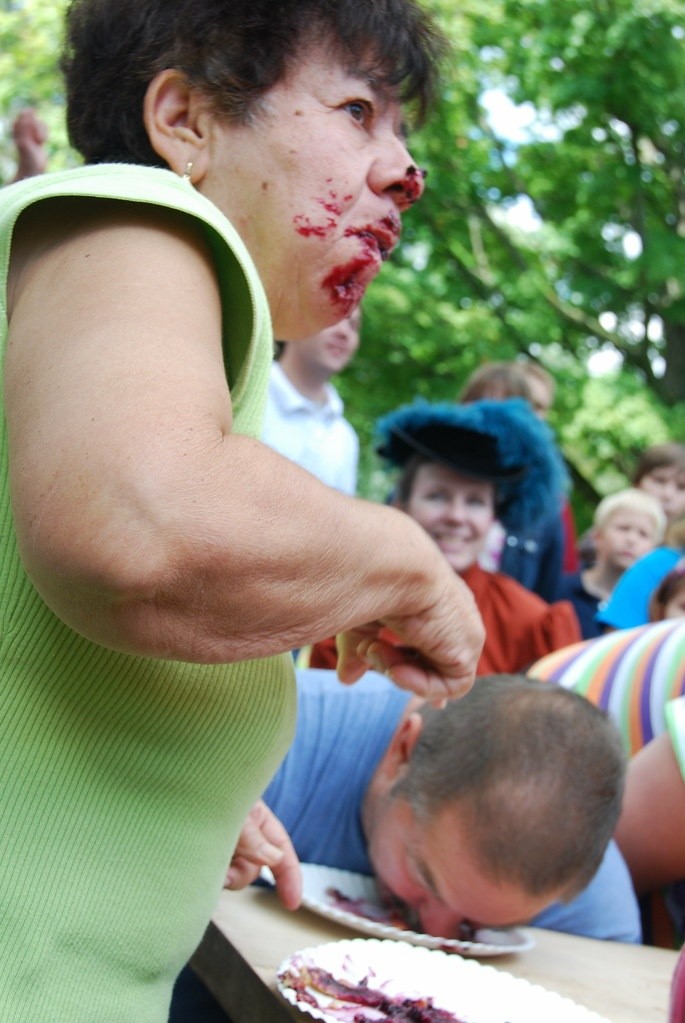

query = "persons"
(312, 359), (684, 950)
(262, 302), (363, 502)
(0, 0), (488, 1023)
(165, 668), (640, 1022)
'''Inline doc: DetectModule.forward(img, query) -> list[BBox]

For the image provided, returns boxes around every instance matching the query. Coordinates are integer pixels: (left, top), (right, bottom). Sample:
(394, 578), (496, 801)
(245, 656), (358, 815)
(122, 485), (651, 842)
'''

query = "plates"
(276, 937), (614, 1023)
(260, 863), (535, 958)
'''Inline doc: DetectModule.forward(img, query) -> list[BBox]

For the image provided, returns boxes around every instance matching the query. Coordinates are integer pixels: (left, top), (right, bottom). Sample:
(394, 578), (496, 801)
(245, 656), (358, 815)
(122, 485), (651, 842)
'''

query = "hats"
(378, 394), (573, 534)
